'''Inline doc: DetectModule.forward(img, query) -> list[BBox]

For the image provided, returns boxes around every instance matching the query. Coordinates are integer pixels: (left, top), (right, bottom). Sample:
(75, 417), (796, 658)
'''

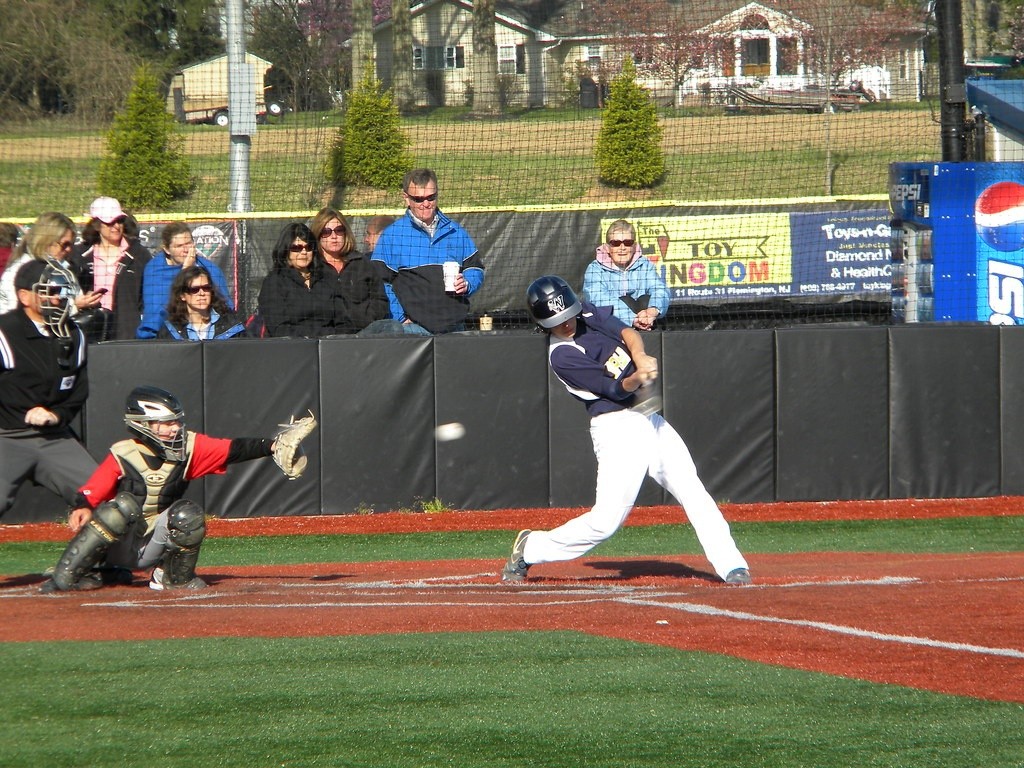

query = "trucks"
(166, 52), (282, 125)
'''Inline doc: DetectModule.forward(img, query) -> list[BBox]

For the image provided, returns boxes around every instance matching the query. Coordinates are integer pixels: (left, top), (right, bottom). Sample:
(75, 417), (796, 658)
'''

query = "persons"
(0, 211), (102, 331)
(309, 208), (394, 335)
(66, 197), (153, 340)
(157, 265), (249, 339)
(582, 220), (671, 332)
(0, 259), (133, 585)
(38, 386), (317, 595)
(137, 222), (234, 339)
(257, 223), (357, 338)
(365, 214), (395, 258)
(502, 275), (751, 585)
(371, 169), (485, 335)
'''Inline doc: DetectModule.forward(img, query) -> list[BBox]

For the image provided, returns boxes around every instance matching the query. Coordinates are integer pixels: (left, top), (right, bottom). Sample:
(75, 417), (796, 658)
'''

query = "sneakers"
(502, 529), (534, 583)
(726, 568), (751, 587)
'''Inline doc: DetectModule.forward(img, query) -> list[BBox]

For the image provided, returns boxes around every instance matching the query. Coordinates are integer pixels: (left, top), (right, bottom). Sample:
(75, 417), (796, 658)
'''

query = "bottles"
(480, 314), (493, 331)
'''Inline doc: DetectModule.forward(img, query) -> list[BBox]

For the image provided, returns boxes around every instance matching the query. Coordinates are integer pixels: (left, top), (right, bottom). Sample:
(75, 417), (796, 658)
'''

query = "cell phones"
(91, 288), (109, 297)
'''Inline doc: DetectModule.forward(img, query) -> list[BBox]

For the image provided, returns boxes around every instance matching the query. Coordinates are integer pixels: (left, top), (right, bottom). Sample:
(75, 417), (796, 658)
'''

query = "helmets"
(527, 276), (582, 328)
(124, 386), (188, 462)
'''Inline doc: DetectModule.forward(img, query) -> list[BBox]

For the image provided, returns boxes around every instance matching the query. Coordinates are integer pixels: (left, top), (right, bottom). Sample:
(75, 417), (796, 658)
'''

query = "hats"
(89, 196), (128, 224)
(14, 260), (72, 291)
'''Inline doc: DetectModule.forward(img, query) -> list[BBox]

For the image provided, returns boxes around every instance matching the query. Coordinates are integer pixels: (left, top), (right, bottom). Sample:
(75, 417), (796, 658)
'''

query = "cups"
(443, 262), (460, 291)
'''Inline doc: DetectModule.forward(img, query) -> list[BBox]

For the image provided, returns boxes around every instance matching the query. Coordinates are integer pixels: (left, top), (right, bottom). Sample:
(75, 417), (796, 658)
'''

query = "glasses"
(287, 242), (313, 252)
(183, 283), (211, 293)
(40, 282), (61, 296)
(98, 217), (125, 226)
(404, 191), (438, 203)
(317, 226), (346, 238)
(607, 239), (635, 247)
(57, 241), (74, 252)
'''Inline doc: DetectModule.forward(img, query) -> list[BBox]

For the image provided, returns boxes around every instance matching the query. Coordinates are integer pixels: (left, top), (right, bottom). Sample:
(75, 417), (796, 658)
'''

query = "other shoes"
(36, 580), (58, 595)
(148, 567), (210, 592)
(96, 561), (132, 585)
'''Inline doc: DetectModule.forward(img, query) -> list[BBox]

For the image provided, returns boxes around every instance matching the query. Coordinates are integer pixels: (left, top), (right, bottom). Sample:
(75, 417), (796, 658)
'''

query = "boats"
(724, 80), (876, 115)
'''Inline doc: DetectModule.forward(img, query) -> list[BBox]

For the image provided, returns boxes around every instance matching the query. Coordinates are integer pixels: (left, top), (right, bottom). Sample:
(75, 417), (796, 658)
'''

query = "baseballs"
(430, 421), (466, 443)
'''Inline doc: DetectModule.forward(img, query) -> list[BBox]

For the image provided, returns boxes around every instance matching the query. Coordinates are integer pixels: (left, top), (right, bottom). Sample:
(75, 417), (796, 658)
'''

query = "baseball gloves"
(271, 416), (319, 481)
